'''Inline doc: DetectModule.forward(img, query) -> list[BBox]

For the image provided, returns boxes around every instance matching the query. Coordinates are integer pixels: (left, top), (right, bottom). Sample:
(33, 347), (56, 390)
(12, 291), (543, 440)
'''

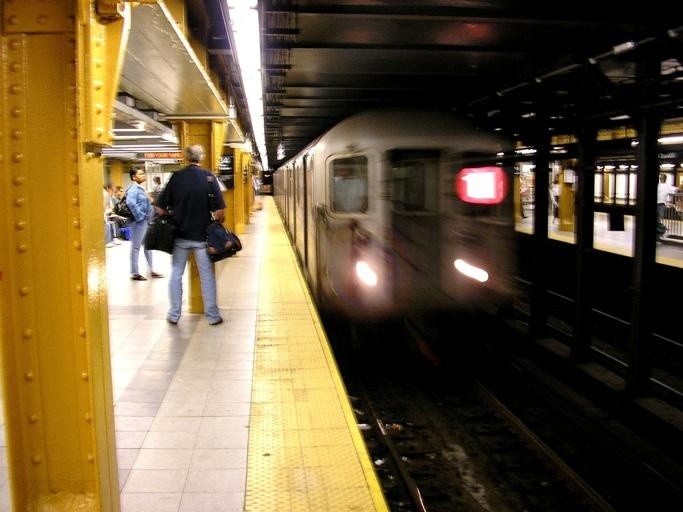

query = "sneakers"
(105, 237), (121, 248)
(131, 272), (164, 281)
(208, 316), (223, 325)
(167, 316), (178, 324)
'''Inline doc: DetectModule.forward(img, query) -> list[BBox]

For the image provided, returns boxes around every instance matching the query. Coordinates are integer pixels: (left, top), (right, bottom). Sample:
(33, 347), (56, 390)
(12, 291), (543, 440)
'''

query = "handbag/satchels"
(143, 215), (175, 255)
(205, 221), (242, 263)
(112, 185), (135, 220)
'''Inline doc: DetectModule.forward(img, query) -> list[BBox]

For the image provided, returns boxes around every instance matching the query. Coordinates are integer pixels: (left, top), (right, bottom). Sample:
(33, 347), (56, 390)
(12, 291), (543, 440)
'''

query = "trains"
(273, 108), (513, 321)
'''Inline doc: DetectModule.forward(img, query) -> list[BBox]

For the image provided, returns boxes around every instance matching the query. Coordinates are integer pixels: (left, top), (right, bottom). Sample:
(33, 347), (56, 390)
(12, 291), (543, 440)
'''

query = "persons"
(329, 159), (368, 213)
(151, 176), (162, 198)
(655, 174), (682, 243)
(102, 181), (120, 247)
(123, 165), (163, 282)
(153, 144), (227, 328)
(110, 185), (132, 241)
(550, 178), (560, 219)
